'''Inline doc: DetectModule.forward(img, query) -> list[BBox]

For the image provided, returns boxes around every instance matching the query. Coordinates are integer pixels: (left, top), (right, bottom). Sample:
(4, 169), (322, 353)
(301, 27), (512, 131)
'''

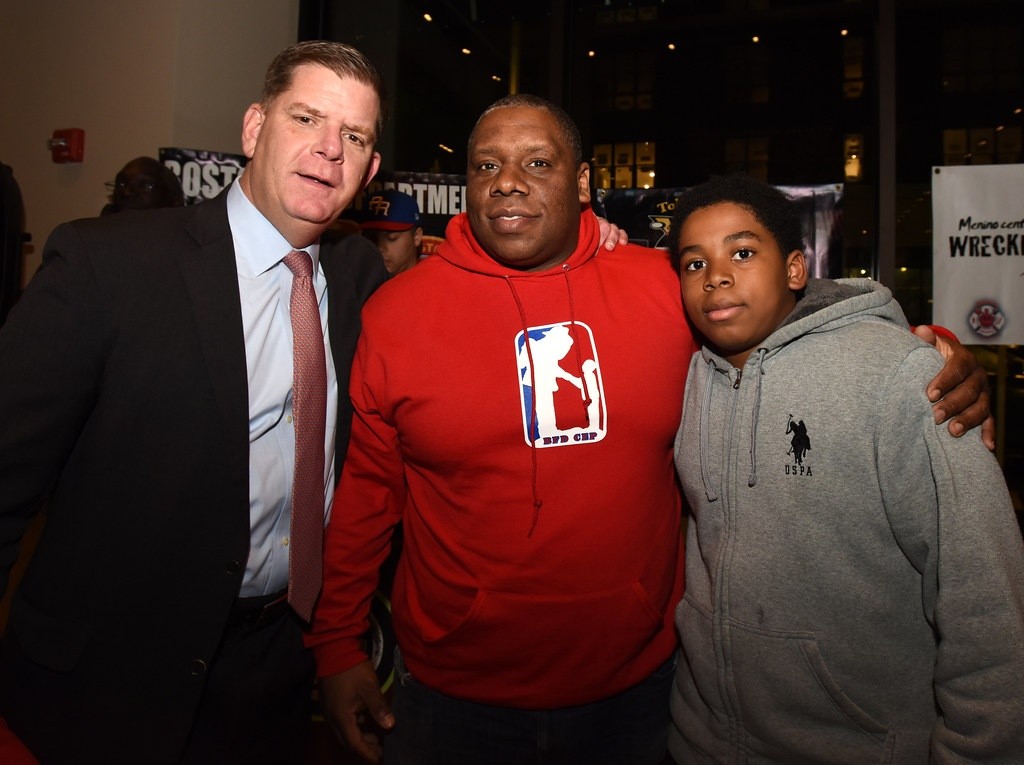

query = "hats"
(352, 190), (420, 230)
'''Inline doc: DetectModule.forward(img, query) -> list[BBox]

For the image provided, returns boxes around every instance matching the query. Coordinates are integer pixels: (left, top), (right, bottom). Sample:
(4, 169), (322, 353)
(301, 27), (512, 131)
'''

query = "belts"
(224, 593), (292, 641)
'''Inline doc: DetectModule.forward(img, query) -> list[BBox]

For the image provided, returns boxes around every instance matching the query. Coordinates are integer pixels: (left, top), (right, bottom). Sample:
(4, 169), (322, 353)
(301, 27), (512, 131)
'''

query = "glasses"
(104, 180), (173, 196)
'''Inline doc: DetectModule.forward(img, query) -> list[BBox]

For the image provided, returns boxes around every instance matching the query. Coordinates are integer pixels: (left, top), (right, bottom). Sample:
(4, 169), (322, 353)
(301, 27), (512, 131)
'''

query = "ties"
(283, 250), (327, 623)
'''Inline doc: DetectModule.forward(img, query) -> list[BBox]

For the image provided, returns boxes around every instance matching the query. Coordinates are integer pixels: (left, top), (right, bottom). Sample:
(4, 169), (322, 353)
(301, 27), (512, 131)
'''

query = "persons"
(106, 156), (183, 212)
(311, 93), (995, 765)
(0, 165), (23, 328)
(661, 174), (1024, 764)
(362, 192), (423, 604)
(0, 40), (628, 765)
